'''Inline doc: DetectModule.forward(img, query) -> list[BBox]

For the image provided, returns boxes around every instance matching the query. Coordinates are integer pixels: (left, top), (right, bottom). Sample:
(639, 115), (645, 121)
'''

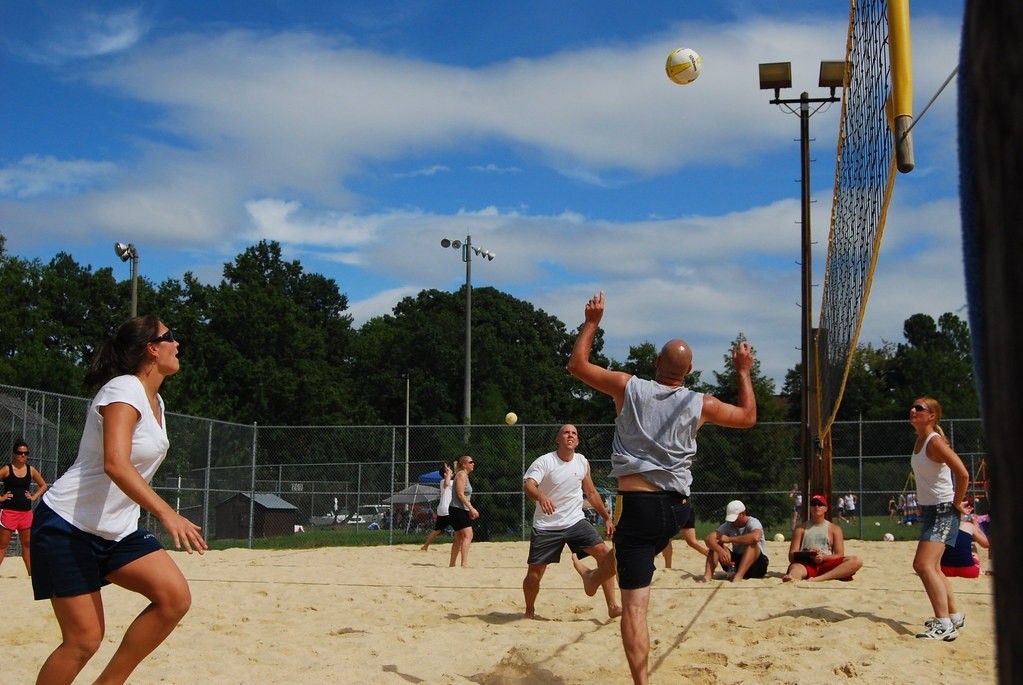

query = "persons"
(782, 495), (863, 583)
(789, 481), (802, 531)
(836, 490), (858, 526)
(421, 456), (479, 568)
(0, 440), (48, 578)
(698, 500), (769, 584)
(941, 494), (990, 578)
(661, 503), (709, 568)
(30, 313), (208, 685)
(569, 290), (757, 685)
(368, 508), (435, 531)
(911, 396), (974, 642)
(889, 494), (922, 524)
(523, 423), (623, 619)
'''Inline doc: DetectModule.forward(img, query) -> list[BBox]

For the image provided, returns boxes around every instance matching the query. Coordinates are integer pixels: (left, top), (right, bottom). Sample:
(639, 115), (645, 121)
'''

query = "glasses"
(810, 501), (822, 507)
(149, 329), (174, 343)
(469, 461), (474, 464)
(17, 450), (29, 456)
(910, 404), (930, 413)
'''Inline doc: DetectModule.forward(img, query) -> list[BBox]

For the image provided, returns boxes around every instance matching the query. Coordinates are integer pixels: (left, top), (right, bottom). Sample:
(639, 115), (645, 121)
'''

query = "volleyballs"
(882, 533), (896, 544)
(906, 521), (912, 526)
(664, 48), (703, 84)
(875, 522), (880, 527)
(774, 532), (786, 541)
(505, 412), (517, 425)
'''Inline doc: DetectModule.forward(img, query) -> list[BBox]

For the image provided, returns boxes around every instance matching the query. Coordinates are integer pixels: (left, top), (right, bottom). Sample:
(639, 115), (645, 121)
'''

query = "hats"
(811, 495), (827, 505)
(725, 500), (746, 522)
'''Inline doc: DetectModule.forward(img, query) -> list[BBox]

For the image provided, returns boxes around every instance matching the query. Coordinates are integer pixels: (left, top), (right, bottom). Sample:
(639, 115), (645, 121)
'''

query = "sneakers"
(916, 614), (965, 642)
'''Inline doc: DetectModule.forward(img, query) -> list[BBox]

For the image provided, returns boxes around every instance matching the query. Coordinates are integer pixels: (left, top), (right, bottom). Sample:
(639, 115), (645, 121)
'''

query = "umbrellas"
(384, 484), (441, 503)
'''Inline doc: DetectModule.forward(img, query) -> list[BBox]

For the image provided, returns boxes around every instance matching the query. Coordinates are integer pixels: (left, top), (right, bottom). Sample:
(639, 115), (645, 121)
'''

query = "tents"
(405, 470), (443, 536)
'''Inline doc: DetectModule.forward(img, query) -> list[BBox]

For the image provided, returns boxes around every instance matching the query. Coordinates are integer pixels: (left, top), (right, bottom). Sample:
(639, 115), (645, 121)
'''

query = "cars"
(319, 505), (365, 527)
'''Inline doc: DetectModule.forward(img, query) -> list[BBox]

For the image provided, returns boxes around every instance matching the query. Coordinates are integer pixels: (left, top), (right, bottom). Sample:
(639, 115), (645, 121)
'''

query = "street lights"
(441, 235), (495, 443)
(759, 61), (850, 502)
(114, 241), (138, 317)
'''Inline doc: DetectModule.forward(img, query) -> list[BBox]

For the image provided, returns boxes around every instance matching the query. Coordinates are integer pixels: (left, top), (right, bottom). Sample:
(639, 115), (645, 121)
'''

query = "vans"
(357, 505), (391, 525)
